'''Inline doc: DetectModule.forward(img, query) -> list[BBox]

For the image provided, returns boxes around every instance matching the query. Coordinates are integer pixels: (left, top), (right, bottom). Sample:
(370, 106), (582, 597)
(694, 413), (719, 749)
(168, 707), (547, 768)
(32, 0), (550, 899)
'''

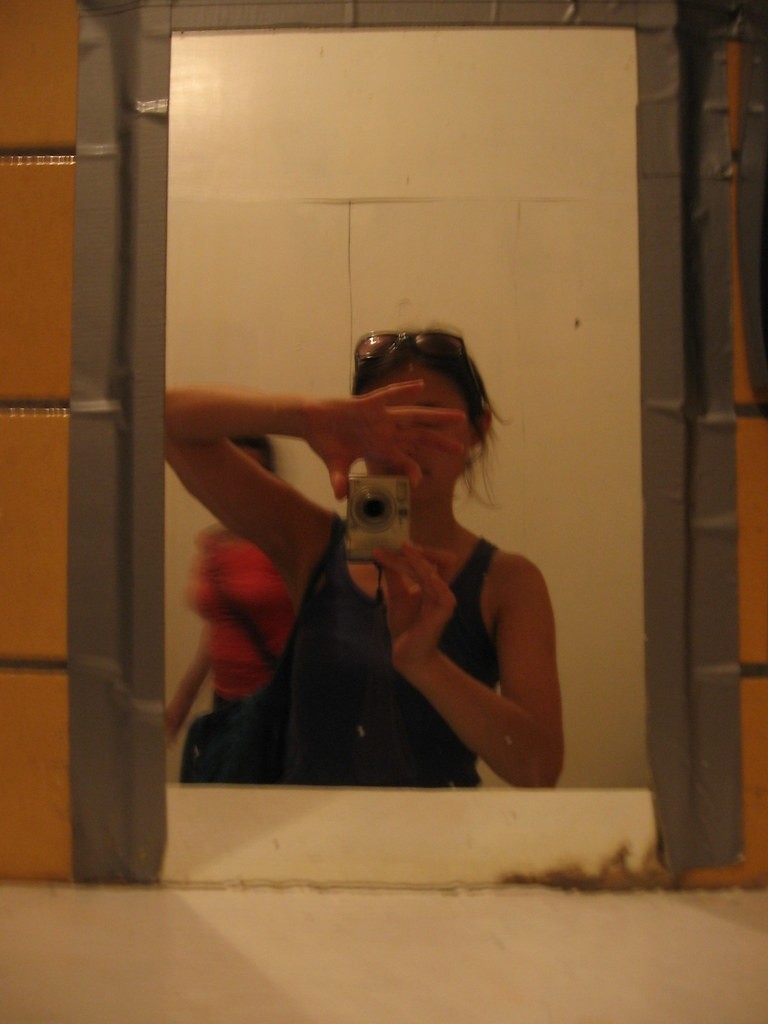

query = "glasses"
(354, 330), (481, 409)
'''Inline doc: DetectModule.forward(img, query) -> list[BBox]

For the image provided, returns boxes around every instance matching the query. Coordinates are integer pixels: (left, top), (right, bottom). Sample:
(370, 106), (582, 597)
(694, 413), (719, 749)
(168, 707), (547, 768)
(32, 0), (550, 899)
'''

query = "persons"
(163, 434), (296, 783)
(164, 329), (566, 789)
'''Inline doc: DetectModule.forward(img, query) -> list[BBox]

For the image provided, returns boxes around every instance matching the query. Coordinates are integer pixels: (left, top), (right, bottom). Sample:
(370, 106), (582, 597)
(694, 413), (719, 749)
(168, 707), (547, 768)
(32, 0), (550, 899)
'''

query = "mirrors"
(69, 0), (746, 891)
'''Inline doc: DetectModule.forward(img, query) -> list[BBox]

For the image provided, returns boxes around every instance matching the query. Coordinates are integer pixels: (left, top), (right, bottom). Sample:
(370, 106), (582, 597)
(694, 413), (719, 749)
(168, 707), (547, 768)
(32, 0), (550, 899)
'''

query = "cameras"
(343, 474), (411, 564)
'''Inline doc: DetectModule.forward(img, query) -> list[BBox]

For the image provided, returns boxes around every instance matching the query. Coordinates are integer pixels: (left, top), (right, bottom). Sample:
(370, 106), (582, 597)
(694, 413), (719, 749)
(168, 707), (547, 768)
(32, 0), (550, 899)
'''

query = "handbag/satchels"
(179, 690), (290, 784)
